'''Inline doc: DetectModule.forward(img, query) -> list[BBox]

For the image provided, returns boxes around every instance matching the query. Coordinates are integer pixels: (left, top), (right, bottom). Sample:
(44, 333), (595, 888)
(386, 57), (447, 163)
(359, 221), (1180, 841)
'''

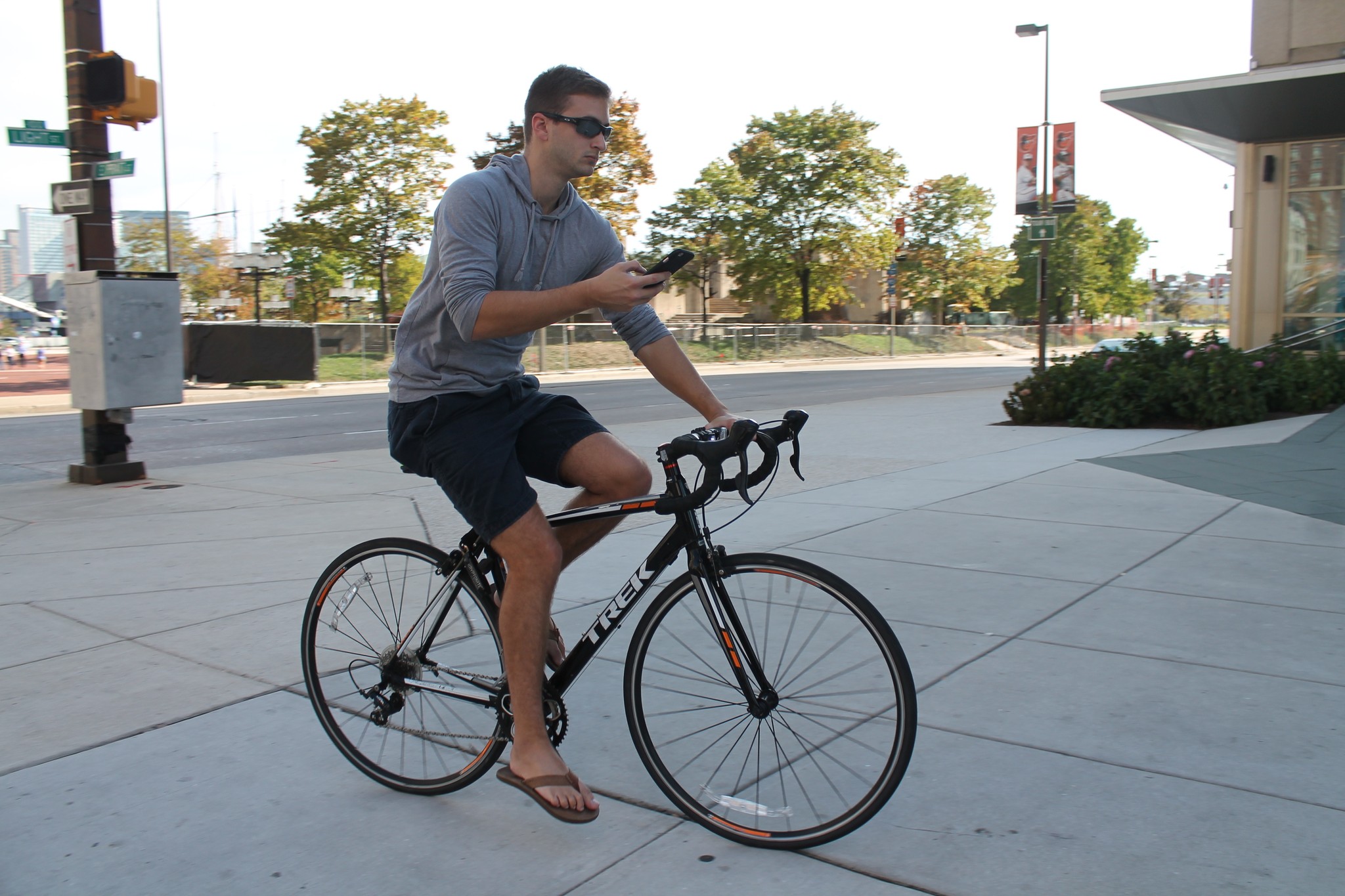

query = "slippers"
(495, 764), (599, 825)
(492, 582), (567, 673)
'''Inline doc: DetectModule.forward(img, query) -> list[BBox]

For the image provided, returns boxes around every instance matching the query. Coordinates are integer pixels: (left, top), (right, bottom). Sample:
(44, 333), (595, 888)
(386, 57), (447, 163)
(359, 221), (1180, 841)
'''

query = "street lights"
(1015, 24), (1049, 370)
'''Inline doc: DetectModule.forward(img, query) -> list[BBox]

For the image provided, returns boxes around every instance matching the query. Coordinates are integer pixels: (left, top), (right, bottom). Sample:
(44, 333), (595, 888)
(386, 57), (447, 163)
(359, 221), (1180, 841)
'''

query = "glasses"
(533, 107), (614, 140)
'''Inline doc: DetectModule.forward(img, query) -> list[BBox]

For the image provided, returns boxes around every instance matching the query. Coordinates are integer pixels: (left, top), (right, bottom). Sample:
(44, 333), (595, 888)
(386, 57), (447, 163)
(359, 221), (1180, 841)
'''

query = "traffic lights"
(84, 51), (140, 107)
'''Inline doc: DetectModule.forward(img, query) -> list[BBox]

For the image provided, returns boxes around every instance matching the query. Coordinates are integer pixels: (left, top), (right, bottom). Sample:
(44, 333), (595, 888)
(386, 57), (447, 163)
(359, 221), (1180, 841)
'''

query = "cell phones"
(641, 249), (695, 289)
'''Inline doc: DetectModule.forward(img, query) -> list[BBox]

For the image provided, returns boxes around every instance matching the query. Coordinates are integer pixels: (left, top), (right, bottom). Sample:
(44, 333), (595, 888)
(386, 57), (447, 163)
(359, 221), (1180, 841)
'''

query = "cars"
(1088, 333), (1231, 361)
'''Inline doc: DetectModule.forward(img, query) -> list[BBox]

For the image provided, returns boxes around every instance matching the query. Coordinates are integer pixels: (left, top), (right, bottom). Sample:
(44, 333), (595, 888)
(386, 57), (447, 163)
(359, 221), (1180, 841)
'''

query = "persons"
(37, 349), (46, 366)
(6, 344), (14, 363)
(17, 340), (25, 361)
(387, 64), (756, 824)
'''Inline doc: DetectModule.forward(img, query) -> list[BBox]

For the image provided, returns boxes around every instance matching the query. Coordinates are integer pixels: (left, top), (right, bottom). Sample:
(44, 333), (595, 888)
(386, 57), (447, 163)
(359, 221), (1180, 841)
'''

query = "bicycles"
(300, 409), (918, 850)
